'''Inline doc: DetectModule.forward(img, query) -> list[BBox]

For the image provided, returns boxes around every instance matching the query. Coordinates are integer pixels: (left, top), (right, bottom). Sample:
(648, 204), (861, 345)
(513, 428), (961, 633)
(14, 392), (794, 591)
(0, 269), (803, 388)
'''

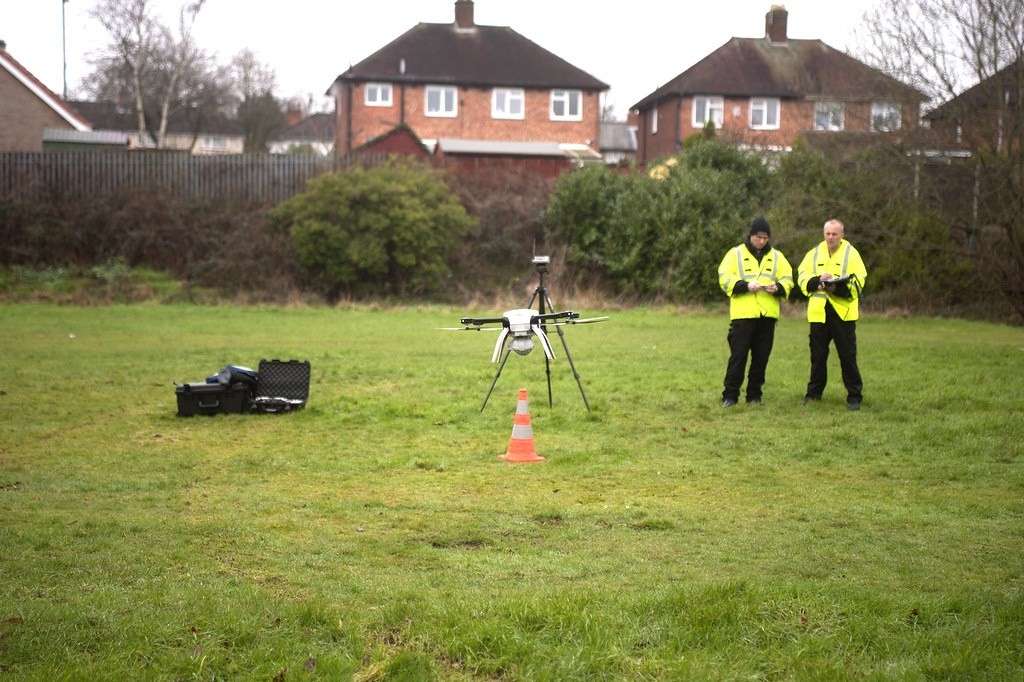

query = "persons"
(796, 219), (868, 410)
(718, 216), (795, 410)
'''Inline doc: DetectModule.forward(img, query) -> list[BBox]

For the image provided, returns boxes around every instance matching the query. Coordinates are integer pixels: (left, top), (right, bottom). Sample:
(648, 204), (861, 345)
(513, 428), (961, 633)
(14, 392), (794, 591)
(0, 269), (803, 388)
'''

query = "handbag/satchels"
(206, 363), (256, 392)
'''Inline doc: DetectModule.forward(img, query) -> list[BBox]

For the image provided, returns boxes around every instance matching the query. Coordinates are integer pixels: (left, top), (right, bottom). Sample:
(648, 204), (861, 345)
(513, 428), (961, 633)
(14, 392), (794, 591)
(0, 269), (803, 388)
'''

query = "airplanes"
(436, 309), (610, 362)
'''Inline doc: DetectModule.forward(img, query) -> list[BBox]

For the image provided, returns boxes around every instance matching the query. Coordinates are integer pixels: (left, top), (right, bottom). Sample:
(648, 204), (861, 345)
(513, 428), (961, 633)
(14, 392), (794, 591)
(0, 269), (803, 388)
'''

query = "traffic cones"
(496, 388), (547, 463)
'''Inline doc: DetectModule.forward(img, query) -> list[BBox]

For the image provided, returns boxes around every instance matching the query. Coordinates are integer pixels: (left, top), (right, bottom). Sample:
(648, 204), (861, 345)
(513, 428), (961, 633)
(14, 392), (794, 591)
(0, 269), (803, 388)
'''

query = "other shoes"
(803, 395), (820, 406)
(746, 398), (761, 404)
(723, 398), (736, 408)
(848, 401), (860, 411)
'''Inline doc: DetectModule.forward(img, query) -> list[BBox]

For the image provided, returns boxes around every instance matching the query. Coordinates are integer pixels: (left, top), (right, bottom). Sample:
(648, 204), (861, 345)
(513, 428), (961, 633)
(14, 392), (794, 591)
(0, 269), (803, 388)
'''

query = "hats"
(750, 217), (770, 238)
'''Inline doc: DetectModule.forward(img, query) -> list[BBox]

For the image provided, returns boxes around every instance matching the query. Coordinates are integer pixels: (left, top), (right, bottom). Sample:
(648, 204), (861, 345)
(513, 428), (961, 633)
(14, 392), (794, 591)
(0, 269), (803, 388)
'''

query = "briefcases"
(250, 359), (311, 414)
(175, 380), (248, 415)
